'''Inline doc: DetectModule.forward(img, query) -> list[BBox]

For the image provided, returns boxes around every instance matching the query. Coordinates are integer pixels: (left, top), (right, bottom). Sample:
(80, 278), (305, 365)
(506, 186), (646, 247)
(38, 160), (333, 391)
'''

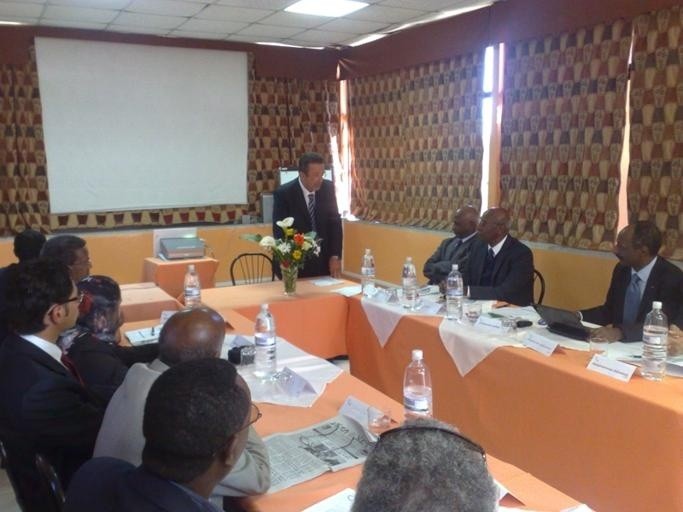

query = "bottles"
(402, 348), (433, 421)
(360, 248), (372, 293)
(399, 256), (415, 307)
(182, 267), (202, 308)
(639, 301), (669, 382)
(445, 262), (460, 321)
(253, 303), (275, 384)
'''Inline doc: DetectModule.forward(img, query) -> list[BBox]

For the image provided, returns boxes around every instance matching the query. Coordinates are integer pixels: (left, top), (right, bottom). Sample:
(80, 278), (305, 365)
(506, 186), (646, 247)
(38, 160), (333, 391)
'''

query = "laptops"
(533, 303), (603, 343)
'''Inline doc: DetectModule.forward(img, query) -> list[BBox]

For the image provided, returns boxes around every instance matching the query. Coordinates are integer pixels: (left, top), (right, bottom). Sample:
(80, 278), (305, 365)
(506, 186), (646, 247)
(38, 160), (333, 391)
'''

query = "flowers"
(239, 218), (326, 289)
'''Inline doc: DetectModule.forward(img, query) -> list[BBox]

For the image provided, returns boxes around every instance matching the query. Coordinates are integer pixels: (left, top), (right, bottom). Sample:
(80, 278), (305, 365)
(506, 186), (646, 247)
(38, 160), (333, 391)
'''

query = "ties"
(622, 274), (641, 325)
(61, 351), (86, 388)
(451, 241), (461, 253)
(309, 194), (317, 232)
(480, 249), (494, 287)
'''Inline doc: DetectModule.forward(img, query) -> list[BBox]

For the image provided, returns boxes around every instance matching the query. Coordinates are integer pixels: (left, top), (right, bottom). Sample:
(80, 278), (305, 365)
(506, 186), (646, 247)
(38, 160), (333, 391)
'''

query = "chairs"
(0, 445), (67, 511)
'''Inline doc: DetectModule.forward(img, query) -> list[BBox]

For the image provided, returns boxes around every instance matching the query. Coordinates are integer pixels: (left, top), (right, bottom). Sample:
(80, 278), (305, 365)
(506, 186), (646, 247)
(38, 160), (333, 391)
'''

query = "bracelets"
(331, 256), (341, 260)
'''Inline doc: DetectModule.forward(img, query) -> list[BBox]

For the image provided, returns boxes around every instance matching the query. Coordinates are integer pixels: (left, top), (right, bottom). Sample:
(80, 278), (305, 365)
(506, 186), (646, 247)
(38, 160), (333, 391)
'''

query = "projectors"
(161, 237), (205, 259)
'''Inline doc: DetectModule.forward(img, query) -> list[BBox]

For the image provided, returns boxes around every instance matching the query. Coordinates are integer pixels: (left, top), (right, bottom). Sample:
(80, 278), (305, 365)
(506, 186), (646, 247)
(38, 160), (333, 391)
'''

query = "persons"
(571, 221), (683, 343)
(348, 416), (501, 512)
(665, 319), (682, 357)
(91, 306), (269, 512)
(58, 355), (254, 512)
(422, 205), (488, 296)
(1, 230), (165, 485)
(272, 151), (344, 281)
(436, 207), (534, 306)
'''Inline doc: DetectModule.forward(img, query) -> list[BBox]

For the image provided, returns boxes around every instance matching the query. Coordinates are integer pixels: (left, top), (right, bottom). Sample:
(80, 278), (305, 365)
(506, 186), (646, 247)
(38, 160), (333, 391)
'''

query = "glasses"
(47, 293), (85, 314)
(212, 404), (261, 458)
(69, 261), (92, 269)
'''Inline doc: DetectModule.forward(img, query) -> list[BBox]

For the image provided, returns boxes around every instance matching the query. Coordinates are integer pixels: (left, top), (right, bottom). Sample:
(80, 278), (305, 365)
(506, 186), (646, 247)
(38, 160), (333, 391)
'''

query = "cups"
(587, 337), (608, 366)
(272, 374), (291, 400)
(367, 407), (391, 433)
(385, 289), (397, 303)
(464, 303), (483, 330)
(504, 316), (517, 341)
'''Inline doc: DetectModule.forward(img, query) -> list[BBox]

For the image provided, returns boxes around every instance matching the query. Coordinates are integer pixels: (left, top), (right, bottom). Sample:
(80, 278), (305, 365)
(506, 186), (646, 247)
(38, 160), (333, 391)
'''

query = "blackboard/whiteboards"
(33, 38), (247, 213)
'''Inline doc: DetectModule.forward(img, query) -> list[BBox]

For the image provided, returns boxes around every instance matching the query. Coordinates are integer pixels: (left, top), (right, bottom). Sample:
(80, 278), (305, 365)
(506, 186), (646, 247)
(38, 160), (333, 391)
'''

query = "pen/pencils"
(152, 327), (155, 335)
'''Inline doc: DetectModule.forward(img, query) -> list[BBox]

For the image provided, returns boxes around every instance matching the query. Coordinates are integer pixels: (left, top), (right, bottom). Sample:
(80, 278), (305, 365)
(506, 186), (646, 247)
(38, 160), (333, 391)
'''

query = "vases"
(280, 266), (297, 293)
